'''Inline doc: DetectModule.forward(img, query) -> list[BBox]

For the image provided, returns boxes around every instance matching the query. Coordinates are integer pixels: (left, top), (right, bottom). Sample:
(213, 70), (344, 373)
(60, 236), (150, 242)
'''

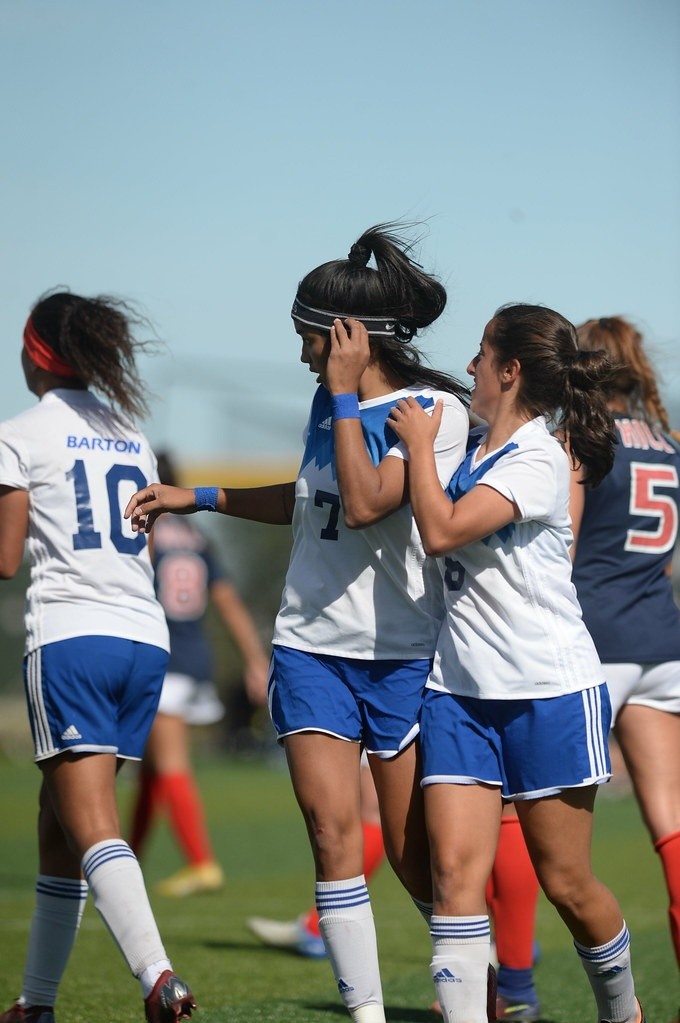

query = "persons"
(128, 449), (273, 898)
(247, 740), (545, 980)
(123, 211), (540, 1023)
(384, 301), (647, 1023)
(0, 292), (196, 1023)
(485, 316), (680, 1023)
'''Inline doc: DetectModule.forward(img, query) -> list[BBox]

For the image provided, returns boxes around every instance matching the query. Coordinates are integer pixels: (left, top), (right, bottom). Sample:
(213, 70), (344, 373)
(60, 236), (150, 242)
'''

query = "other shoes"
(496, 994), (539, 1023)
(246, 917), (328, 958)
(151, 863), (223, 897)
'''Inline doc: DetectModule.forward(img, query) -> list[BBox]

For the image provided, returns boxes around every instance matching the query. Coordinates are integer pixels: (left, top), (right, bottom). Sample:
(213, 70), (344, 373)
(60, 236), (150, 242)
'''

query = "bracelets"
(194, 487), (219, 512)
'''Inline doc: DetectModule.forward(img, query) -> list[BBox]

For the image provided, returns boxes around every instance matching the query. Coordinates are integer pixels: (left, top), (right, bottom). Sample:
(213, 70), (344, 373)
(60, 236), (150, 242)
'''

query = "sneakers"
(145, 969), (197, 1023)
(0, 1003), (54, 1023)
